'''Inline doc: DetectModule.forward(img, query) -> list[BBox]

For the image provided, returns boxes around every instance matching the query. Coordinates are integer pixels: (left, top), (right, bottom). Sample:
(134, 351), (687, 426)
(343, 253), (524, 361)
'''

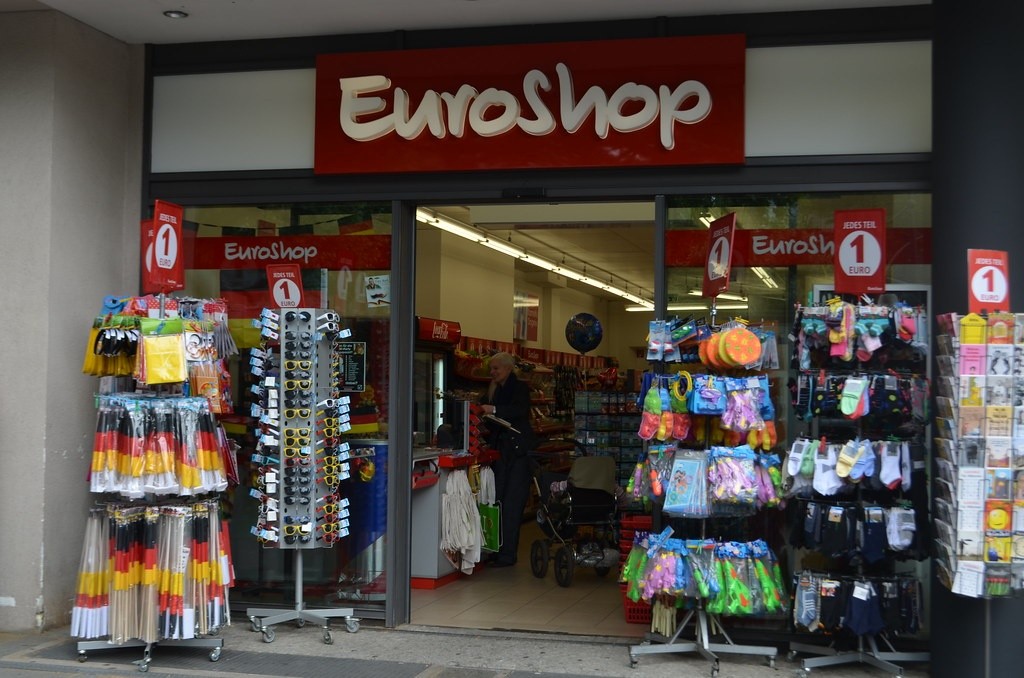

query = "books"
(932, 313), (1024, 598)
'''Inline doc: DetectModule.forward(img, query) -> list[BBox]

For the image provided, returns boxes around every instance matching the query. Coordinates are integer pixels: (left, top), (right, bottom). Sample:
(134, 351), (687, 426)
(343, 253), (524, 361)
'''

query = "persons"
(480, 352), (531, 568)
(989, 546), (1003, 561)
(966, 439), (974, 464)
(992, 379), (1007, 403)
(367, 278), (381, 290)
(959, 538), (968, 556)
(991, 349), (1010, 375)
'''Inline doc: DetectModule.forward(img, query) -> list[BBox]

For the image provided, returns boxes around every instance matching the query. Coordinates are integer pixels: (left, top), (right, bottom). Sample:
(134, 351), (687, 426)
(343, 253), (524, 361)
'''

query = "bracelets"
(492, 406), (496, 415)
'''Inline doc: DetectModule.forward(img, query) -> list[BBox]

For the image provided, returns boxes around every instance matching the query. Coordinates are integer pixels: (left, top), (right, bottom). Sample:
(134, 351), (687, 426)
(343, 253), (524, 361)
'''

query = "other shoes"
(483, 557), (515, 568)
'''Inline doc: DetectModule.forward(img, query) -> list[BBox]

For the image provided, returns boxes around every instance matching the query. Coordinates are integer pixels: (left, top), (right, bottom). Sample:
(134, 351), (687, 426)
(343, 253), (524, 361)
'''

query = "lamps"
(414, 205), (778, 311)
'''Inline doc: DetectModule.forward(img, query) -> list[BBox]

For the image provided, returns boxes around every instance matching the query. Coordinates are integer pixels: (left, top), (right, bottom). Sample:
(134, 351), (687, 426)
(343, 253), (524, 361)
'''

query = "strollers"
(529, 437), (628, 588)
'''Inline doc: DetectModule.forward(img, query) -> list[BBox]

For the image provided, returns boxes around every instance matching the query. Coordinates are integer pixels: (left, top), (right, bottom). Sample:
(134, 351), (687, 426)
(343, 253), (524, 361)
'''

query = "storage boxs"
(621, 516), (663, 625)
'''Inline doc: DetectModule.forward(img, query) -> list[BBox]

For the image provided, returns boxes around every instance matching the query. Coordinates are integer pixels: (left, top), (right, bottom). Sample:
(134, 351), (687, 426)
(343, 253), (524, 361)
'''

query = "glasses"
(256, 310), (342, 550)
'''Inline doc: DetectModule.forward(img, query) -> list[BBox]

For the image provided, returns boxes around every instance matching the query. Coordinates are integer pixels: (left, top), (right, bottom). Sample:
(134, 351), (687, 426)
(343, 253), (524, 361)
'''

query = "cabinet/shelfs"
(463, 339), (649, 515)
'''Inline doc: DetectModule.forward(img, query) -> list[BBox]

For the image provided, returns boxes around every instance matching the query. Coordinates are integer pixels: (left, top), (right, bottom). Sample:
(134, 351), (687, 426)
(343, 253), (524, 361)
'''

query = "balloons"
(565, 313), (602, 353)
